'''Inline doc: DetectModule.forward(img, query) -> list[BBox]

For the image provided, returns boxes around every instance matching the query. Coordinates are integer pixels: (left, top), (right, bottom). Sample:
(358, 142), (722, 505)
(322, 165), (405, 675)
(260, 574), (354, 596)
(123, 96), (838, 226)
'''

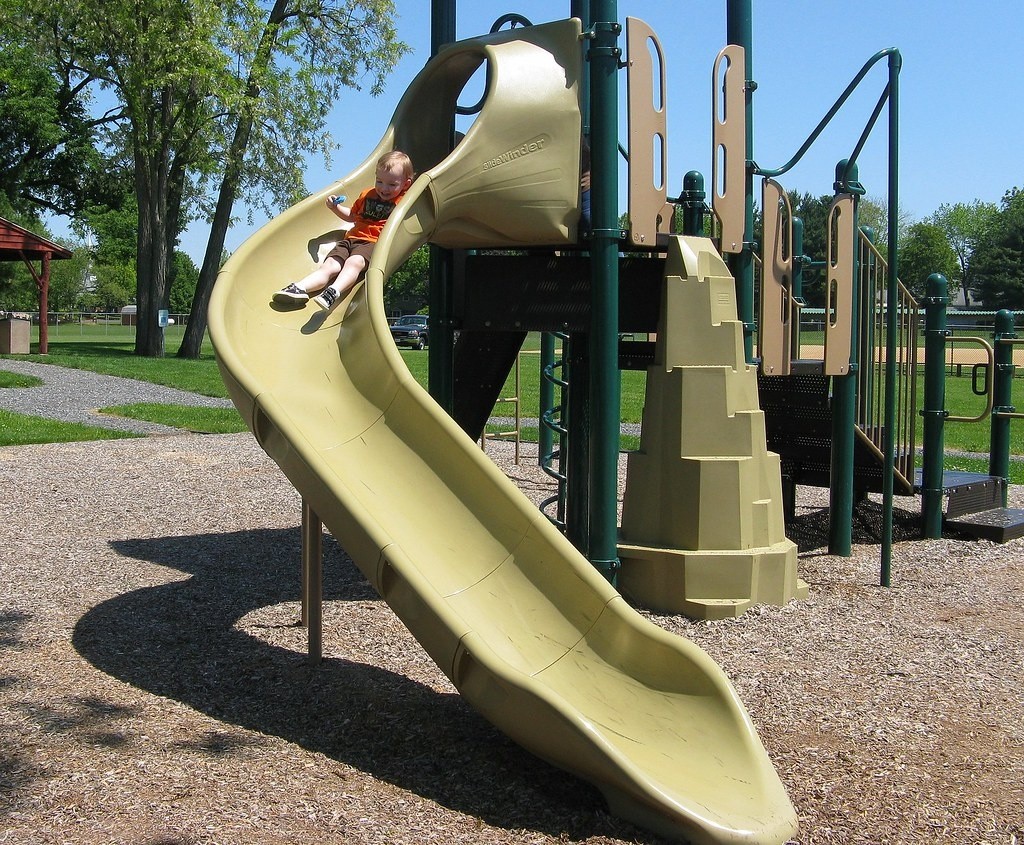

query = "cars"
(389, 316), (431, 350)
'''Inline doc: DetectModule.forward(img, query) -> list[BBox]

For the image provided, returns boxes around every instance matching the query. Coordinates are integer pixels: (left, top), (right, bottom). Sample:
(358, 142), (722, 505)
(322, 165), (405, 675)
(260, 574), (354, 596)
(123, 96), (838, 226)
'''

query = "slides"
(209, 15), (807, 845)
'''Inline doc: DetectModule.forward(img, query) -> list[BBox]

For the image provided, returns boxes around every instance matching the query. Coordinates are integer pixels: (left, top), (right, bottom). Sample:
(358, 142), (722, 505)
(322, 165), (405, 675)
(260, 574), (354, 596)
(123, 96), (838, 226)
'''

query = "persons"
(579, 134), (592, 230)
(271, 152), (415, 312)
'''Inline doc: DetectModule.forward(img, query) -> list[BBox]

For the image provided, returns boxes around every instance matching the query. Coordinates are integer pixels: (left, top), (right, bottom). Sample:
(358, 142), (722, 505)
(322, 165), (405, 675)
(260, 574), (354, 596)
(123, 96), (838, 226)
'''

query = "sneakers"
(314, 287), (341, 313)
(273, 282), (309, 305)
